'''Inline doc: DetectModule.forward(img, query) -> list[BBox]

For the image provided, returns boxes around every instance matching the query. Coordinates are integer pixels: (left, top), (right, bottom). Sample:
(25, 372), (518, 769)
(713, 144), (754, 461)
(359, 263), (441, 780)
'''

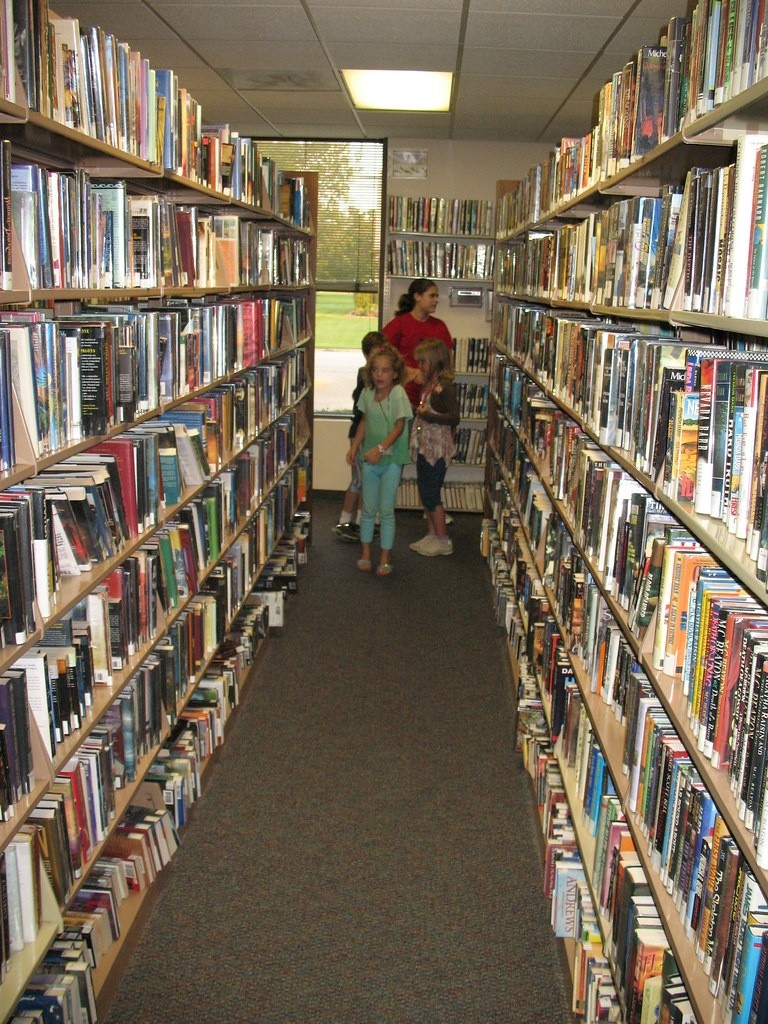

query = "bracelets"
(379, 445), (385, 454)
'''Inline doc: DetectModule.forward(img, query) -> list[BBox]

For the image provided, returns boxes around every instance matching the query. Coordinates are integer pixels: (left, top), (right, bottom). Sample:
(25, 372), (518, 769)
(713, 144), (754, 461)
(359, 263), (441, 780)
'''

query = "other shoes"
(423, 512), (454, 524)
(357, 560), (371, 570)
(376, 561), (394, 576)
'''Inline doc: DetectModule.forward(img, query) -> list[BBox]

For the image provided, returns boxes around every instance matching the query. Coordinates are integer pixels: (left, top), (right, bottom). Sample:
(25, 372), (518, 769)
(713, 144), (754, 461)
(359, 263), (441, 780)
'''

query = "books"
(389, 195), (494, 510)
(0, 0), (312, 1024)
(482, 0), (768, 1024)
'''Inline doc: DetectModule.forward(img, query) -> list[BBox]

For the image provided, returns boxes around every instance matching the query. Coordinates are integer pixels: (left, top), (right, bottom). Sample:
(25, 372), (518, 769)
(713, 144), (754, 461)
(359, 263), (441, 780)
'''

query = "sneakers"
(409, 533), (452, 556)
(332, 522), (380, 541)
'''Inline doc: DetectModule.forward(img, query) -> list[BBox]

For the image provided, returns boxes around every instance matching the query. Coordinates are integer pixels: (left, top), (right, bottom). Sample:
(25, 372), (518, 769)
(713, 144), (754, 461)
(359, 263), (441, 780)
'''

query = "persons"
(331, 280), (460, 575)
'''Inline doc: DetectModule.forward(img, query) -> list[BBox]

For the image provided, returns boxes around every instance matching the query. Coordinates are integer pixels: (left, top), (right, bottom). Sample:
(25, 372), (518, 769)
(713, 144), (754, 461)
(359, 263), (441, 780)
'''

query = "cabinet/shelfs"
(0, 0), (321, 1024)
(378, 0), (768, 1024)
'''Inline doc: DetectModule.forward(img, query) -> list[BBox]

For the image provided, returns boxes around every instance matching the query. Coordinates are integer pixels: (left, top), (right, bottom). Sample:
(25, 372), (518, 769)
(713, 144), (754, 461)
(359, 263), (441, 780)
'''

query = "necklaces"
(376, 388), (390, 424)
